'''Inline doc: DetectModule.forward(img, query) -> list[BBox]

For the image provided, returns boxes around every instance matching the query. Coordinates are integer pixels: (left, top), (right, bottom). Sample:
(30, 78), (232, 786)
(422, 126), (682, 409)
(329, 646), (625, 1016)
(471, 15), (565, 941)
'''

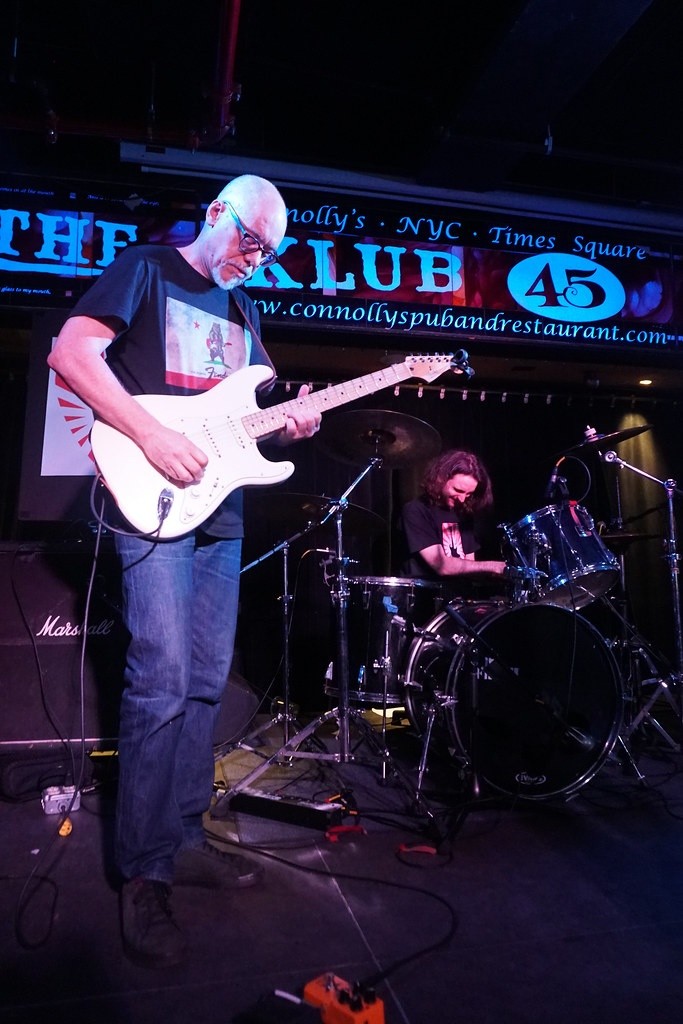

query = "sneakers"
(173, 828), (264, 889)
(120, 877), (188, 967)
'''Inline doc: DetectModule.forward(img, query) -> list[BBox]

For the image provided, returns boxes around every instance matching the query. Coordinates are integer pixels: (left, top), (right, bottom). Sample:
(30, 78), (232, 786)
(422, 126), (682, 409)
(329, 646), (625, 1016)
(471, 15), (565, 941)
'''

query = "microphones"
(544, 466), (560, 499)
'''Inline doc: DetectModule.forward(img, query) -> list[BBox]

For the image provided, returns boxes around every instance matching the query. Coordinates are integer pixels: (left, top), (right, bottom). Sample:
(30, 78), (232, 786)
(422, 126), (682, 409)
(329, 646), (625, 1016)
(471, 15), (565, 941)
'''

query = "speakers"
(10, 315), (129, 534)
(0, 524), (132, 754)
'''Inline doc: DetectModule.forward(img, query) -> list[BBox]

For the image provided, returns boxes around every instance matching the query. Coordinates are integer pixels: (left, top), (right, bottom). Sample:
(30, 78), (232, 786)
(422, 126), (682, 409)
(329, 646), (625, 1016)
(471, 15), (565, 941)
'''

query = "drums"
(323, 575), (446, 705)
(499, 501), (622, 612)
(398, 597), (626, 803)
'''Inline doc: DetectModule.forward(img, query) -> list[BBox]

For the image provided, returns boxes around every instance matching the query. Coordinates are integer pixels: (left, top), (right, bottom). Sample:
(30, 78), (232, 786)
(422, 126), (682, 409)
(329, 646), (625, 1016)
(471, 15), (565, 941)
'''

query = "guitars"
(87, 349), (476, 544)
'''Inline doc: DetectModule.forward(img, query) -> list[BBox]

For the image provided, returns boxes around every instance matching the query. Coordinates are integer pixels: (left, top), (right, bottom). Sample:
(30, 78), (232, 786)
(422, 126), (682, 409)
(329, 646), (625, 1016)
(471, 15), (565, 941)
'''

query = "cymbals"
(536, 422), (656, 467)
(258, 493), (387, 540)
(313, 409), (443, 472)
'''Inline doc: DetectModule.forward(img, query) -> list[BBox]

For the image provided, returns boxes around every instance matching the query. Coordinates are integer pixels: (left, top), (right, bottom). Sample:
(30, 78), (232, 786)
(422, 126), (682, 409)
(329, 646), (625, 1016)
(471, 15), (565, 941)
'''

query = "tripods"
(211, 456), (452, 858)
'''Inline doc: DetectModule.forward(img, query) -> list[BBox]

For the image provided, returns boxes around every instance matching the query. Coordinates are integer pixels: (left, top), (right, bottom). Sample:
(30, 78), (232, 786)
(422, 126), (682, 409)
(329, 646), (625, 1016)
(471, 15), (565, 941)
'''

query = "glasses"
(220, 201), (280, 268)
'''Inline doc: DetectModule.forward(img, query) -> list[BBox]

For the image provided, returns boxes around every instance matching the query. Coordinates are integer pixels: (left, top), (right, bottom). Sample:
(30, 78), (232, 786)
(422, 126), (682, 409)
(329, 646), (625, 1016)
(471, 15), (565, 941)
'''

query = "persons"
(46, 172), (324, 971)
(400, 450), (507, 630)
